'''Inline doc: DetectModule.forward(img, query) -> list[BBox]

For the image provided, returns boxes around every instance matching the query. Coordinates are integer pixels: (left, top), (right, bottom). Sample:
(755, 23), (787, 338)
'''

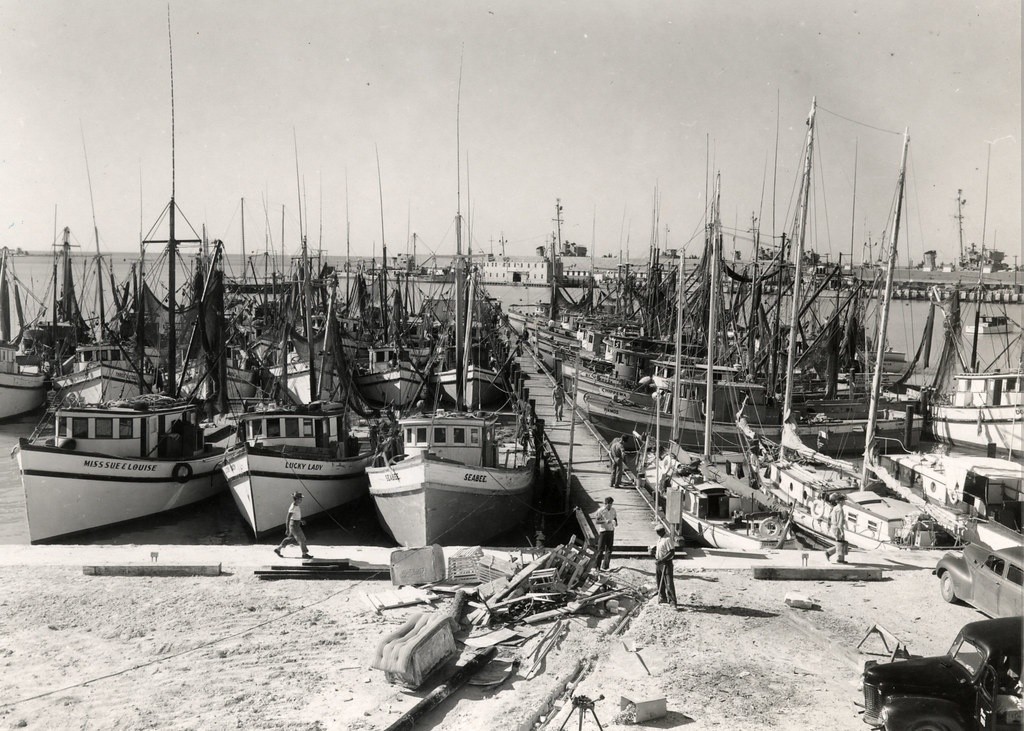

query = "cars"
(864, 617), (1023, 731)
(933, 538), (1023, 617)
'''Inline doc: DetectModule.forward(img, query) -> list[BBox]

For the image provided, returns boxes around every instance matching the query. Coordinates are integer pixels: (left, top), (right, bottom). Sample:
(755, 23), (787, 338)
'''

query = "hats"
(653, 524), (664, 530)
(291, 491), (304, 498)
(836, 495), (847, 500)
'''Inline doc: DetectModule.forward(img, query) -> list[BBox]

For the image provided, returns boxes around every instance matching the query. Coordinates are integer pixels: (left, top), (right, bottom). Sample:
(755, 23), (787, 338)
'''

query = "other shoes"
(825, 552), (830, 561)
(659, 598), (667, 603)
(556, 417), (562, 422)
(609, 485), (615, 487)
(670, 605), (678, 611)
(274, 549), (283, 557)
(615, 486), (623, 488)
(836, 560), (848, 564)
(302, 554), (313, 558)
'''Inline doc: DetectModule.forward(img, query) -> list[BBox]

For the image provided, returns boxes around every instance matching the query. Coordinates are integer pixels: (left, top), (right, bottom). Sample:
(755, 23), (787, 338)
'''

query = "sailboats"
(53, 120), (153, 407)
(508, 102), (937, 450)
(162, 224), (255, 415)
(427, 151), (502, 411)
(363, 42), (546, 546)
(15, 2), (226, 545)
(633, 172), (796, 550)
(0, 247), (46, 425)
(926, 143), (1024, 459)
(353, 143), (426, 407)
(221, 124), (379, 539)
(735, 97), (934, 551)
(196, 198), (494, 356)
(268, 136), (347, 408)
(34, 226), (90, 378)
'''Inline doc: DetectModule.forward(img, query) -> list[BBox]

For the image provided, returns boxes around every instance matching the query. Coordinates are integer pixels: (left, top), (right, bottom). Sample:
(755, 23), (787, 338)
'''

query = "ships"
(330, 232), (474, 288)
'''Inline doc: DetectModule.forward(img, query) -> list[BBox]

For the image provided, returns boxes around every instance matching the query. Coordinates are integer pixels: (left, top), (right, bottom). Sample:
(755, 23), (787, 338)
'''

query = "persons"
(648, 524), (679, 610)
(552, 381), (566, 422)
(825, 495), (849, 564)
(492, 302), (529, 357)
(594, 496), (618, 572)
(273, 492), (314, 559)
(609, 432), (629, 484)
(610, 434), (629, 488)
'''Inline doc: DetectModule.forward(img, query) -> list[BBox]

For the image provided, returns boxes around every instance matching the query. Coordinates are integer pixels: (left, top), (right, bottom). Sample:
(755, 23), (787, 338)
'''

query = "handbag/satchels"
(836, 536), (848, 555)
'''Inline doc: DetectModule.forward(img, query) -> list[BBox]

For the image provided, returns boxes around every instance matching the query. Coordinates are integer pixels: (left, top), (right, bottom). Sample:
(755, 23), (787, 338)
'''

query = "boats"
(966, 315), (1014, 333)
(868, 436), (1022, 545)
